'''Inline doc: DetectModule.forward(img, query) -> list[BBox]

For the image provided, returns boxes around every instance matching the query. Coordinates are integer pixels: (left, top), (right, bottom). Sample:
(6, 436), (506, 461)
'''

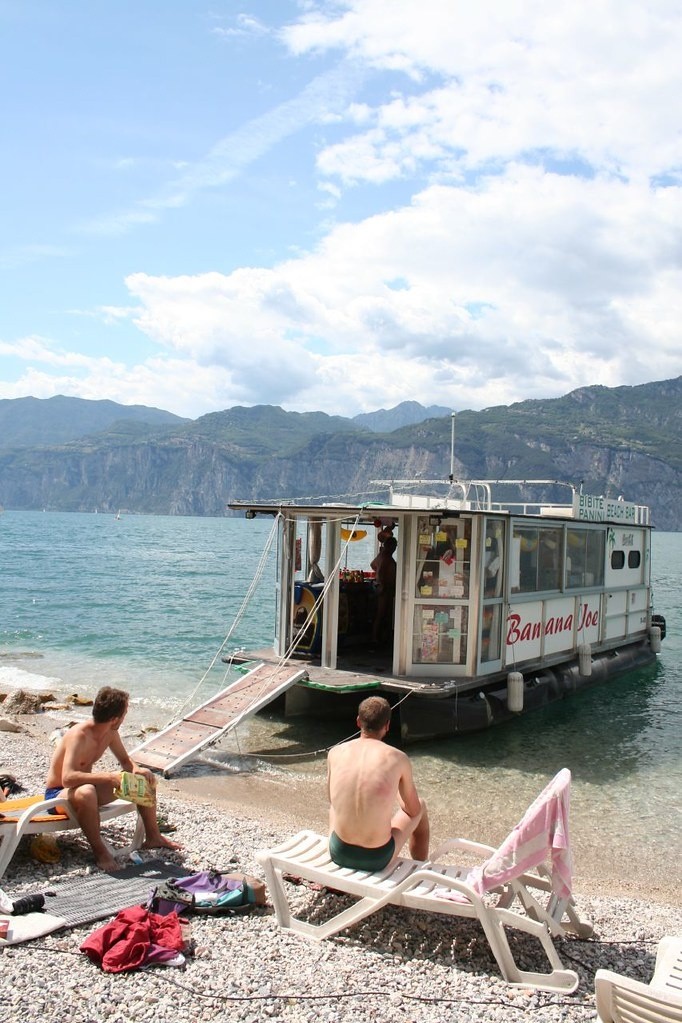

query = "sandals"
(158, 820), (176, 832)
(156, 812), (168, 819)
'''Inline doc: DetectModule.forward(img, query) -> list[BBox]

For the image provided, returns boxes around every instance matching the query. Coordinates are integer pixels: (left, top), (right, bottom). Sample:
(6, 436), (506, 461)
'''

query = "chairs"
(595, 936), (682, 1023)
(0, 777), (150, 885)
(254, 768), (595, 995)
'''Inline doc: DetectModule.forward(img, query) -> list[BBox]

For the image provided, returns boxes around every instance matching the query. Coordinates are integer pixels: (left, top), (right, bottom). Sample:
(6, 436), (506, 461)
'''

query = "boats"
(114, 509), (121, 520)
(221, 411), (665, 743)
(96, 508), (98, 514)
(43, 507), (46, 512)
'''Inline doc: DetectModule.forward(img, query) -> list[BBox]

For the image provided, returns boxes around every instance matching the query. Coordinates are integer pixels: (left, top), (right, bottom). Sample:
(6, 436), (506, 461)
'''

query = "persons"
(371, 537), (397, 650)
(45, 685), (184, 873)
(327, 696), (430, 871)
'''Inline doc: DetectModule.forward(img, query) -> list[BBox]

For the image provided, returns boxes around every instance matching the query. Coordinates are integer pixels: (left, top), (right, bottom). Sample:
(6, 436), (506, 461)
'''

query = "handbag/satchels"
(150, 869), (254, 907)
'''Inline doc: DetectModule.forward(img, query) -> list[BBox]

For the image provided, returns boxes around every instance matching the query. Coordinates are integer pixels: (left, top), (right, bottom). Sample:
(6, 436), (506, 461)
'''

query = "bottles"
(339, 566), (364, 582)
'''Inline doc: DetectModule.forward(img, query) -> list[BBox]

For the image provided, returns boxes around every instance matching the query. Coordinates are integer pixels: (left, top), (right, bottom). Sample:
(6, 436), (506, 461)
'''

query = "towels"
(435, 767), (573, 905)
